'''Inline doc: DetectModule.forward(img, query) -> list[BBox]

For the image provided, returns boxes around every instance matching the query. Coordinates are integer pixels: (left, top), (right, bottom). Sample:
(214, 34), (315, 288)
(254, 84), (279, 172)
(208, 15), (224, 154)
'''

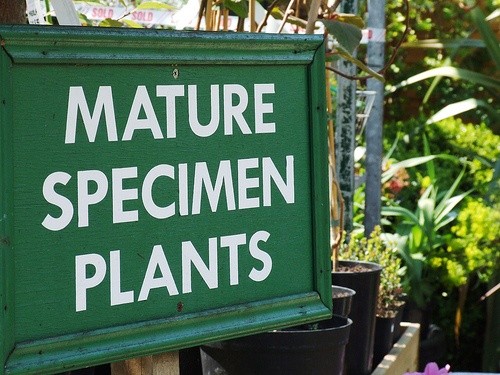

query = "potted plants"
(216, 252), (387, 375)
